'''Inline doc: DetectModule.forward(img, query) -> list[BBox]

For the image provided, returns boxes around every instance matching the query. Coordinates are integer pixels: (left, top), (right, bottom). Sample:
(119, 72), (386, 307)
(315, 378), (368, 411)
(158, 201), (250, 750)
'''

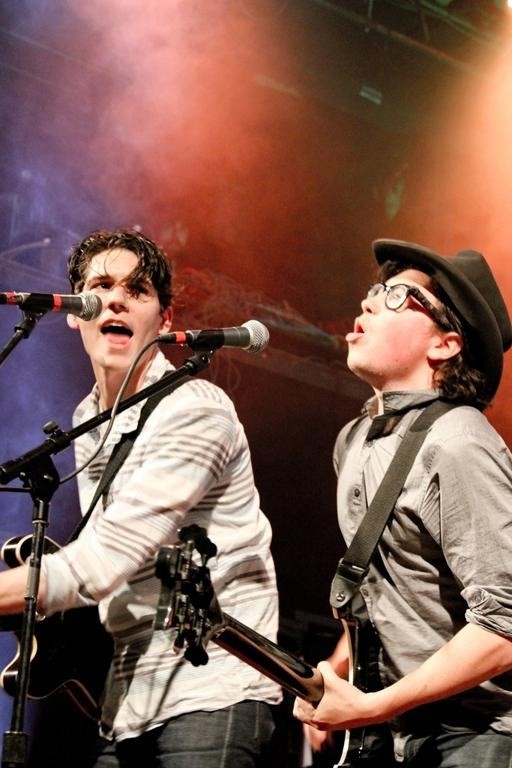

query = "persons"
(292, 238), (512, 767)
(0, 224), (284, 767)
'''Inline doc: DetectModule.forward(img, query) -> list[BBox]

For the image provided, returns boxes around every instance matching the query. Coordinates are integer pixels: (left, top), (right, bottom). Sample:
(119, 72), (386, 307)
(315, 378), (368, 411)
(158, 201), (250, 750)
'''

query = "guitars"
(153, 525), (380, 768)
(2, 533), (107, 720)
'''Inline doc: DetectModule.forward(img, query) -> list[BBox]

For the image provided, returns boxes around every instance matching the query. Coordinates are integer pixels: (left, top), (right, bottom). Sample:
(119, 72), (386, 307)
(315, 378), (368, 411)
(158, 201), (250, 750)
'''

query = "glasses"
(366, 281), (453, 333)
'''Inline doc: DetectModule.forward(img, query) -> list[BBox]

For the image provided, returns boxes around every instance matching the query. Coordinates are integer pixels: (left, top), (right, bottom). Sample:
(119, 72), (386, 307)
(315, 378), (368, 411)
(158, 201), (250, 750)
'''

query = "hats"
(371, 235), (512, 412)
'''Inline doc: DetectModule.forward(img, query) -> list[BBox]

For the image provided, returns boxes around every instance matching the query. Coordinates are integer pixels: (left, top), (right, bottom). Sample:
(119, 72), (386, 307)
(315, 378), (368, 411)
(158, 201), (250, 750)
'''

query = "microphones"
(158, 317), (272, 354)
(0, 287), (102, 320)
